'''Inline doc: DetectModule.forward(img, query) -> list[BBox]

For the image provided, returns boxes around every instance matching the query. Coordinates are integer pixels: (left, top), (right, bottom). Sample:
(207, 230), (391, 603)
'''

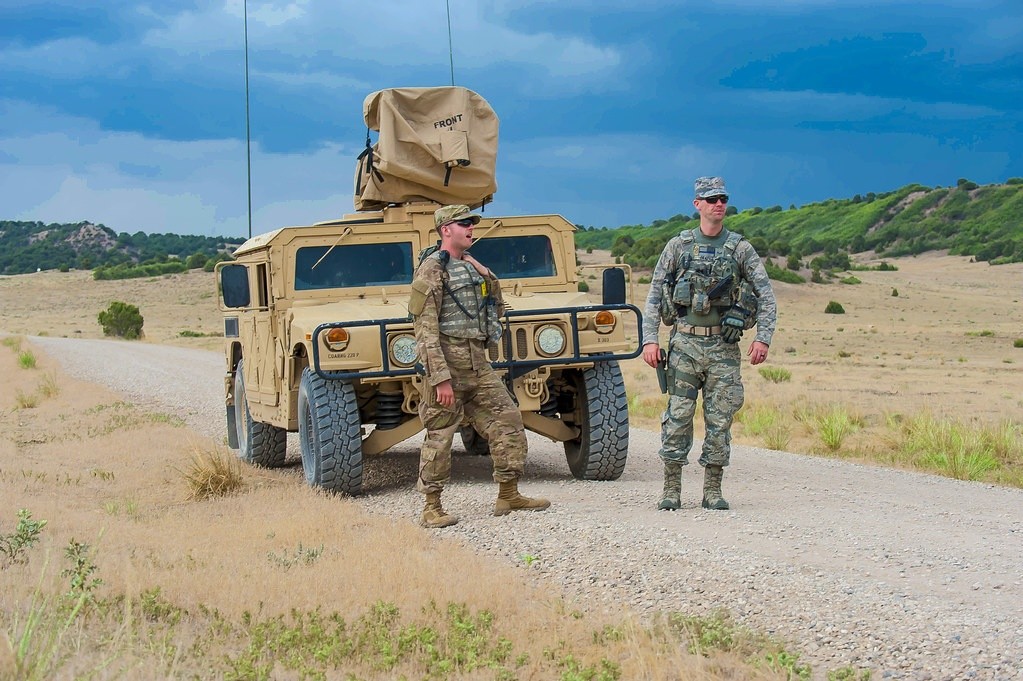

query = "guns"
(414, 362), (436, 408)
(655, 348), (667, 394)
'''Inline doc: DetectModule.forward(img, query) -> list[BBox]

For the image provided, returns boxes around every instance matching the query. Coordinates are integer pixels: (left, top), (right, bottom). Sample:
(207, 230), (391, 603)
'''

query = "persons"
(642, 176), (777, 509)
(408, 205), (551, 527)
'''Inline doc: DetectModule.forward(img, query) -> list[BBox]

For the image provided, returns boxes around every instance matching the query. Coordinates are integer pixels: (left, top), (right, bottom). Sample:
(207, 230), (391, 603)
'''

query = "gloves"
(720, 306), (745, 343)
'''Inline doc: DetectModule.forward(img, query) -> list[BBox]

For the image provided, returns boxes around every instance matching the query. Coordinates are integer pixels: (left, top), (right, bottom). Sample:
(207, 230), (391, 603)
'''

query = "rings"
(761, 354), (764, 356)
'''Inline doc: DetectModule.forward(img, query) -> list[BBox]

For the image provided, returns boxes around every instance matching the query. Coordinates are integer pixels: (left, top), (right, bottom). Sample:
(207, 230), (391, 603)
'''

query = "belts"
(677, 323), (721, 336)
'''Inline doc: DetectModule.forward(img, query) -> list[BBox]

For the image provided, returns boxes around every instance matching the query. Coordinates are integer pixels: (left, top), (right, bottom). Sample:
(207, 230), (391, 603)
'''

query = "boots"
(657, 463), (682, 510)
(419, 491), (458, 528)
(494, 478), (551, 516)
(702, 464), (729, 509)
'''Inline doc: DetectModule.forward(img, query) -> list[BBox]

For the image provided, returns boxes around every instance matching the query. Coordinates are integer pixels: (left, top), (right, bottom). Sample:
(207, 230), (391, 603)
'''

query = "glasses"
(697, 195), (729, 204)
(445, 218), (474, 227)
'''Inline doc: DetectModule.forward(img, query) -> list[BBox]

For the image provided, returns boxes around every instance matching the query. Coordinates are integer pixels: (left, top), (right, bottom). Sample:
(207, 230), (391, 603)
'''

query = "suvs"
(214, 202), (643, 495)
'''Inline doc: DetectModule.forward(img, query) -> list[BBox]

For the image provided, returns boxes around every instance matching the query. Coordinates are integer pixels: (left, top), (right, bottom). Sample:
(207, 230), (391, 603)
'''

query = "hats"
(695, 176), (729, 197)
(434, 205), (480, 229)
